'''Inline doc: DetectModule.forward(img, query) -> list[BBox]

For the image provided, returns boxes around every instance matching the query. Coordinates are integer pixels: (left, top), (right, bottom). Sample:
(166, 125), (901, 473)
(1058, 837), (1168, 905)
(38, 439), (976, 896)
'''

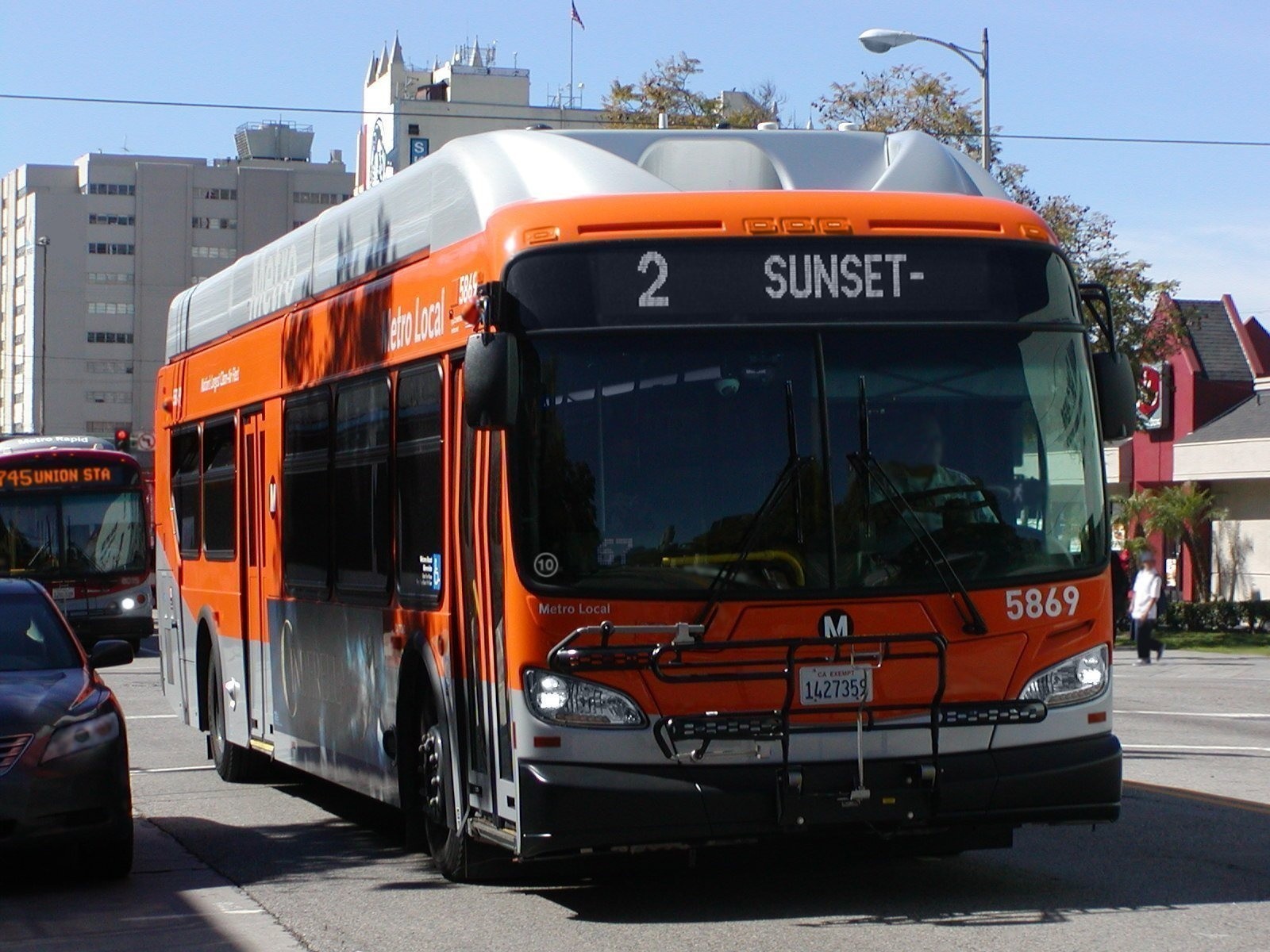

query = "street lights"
(855, 27), (994, 175)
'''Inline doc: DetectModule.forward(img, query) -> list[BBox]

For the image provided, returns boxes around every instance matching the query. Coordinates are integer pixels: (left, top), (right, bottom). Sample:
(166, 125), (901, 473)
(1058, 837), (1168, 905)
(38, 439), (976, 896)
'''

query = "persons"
(869, 418), (999, 530)
(1128, 551), (1165, 666)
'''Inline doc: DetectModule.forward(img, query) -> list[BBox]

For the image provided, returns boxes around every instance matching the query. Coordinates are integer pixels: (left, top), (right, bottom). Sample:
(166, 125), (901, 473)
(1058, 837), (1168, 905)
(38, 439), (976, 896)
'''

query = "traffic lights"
(115, 429), (132, 452)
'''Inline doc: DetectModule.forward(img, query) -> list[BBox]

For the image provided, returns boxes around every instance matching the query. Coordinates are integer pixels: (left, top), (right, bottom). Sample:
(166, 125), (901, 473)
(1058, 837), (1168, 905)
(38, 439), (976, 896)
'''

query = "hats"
(1140, 552), (1155, 563)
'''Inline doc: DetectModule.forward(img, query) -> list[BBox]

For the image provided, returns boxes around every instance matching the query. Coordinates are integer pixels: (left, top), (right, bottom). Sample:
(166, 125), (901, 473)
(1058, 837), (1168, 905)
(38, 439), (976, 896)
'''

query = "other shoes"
(1158, 643), (1164, 660)
(1141, 660), (1151, 666)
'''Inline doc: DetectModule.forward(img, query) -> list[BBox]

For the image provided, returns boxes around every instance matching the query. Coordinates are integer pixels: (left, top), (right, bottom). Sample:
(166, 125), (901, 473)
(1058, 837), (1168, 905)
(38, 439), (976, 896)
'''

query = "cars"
(0, 573), (137, 881)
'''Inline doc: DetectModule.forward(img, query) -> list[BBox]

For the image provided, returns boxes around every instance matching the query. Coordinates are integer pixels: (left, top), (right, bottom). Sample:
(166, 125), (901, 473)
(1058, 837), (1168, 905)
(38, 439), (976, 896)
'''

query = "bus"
(152, 125), (1142, 884)
(0, 433), (156, 649)
